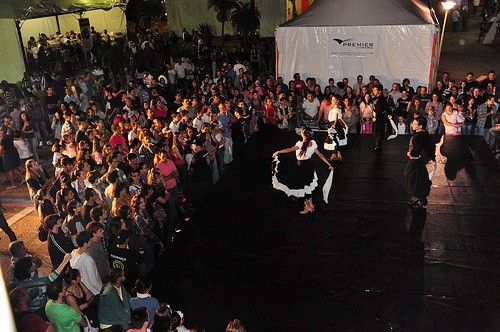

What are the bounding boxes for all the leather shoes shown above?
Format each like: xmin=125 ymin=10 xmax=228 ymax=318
xmin=409 ymin=196 xmax=427 ymax=209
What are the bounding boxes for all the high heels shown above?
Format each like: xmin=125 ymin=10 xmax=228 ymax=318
xmin=300 ymin=205 xmax=314 ymax=214
xmin=326 ymin=155 xmax=343 ymax=161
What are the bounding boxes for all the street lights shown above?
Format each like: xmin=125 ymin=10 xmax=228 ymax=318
xmin=440 ymin=0 xmax=460 ymax=59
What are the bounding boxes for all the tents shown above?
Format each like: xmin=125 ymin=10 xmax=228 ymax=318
xmin=0 ymin=0 xmax=128 ymax=83
xmin=272 ymin=0 xmax=441 ymax=94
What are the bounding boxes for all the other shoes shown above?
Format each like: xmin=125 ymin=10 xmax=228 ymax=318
xmin=371 ymin=145 xmax=382 ymax=151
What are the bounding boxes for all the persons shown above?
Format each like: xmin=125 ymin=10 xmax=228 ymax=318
xmin=271 ymin=127 xmax=335 ymax=215
xmin=0 ymin=0 xmax=500 ymax=332
